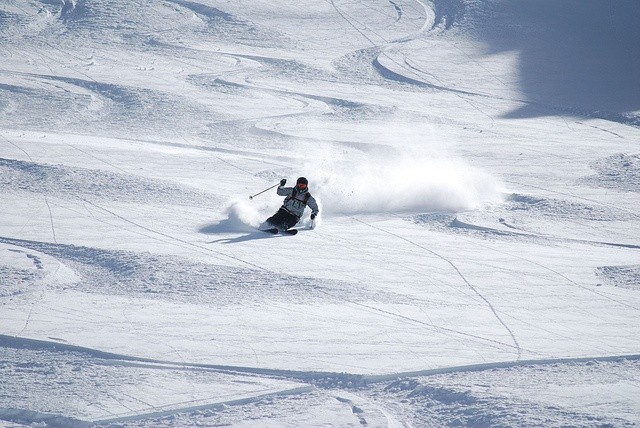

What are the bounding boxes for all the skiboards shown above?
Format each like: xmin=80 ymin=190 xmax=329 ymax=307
xmin=261 ymin=228 xmax=298 ymax=235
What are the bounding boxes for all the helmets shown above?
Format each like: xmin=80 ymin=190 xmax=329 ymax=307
xmin=297 ymin=177 xmax=308 ymax=190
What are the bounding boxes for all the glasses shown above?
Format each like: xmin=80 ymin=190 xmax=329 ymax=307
xmin=297 ymin=183 xmax=306 ymax=189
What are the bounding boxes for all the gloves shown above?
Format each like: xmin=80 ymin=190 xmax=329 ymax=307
xmin=280 ymin=179 xmax=286 ymax=186
xmin=311 ymin=214 xmax=315 ymax=219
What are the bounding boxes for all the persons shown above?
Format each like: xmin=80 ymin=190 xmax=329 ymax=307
xmin=264 ymin=177 xmax=320 ymax=231
